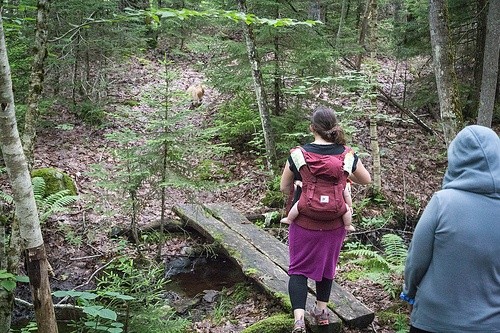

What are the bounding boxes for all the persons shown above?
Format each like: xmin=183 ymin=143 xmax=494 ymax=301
xmin=278 ymin=110 xmax=371 ymax=333
xmin=399 ymin=122 xmax=500 ymax=333
xmin=279 ymin=156 xmax=357 ymax=232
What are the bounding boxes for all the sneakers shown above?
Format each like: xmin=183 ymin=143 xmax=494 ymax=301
xmin=310 ymin=307 xmax=330 ymax=326
xmin=292 ymin=320 xmax=307 ymax=333
